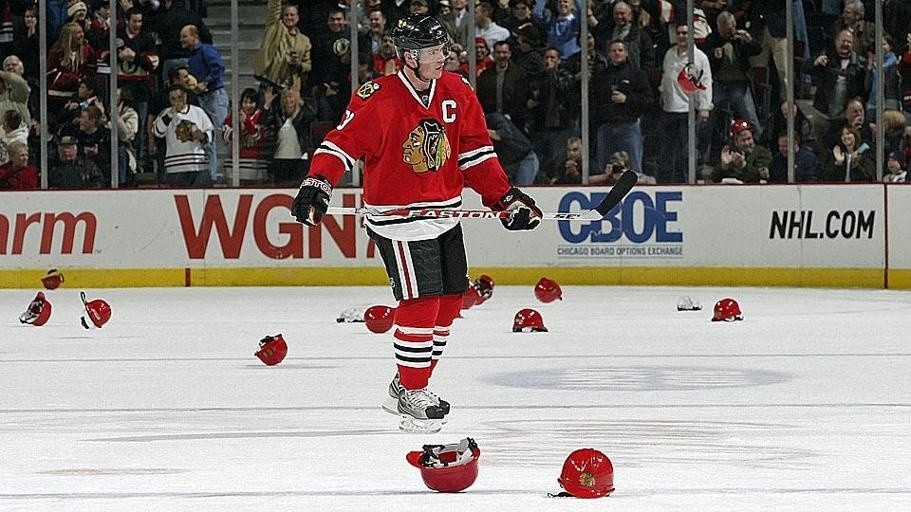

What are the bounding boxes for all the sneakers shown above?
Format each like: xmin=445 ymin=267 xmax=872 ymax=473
xmin=389 ymin=374 xmax=449 ymax=418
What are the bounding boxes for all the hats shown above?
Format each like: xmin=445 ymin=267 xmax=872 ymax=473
xmin=890 ymin=151 xmax=907 ymax=170
xmin=730 ymin=119 xmax=751 ymax=135
xmin=67 ymin=0 xmax=87 ymax=16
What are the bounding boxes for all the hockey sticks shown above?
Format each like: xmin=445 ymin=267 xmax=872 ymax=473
xmin=325 ymin=171 xmax=638 ymax=221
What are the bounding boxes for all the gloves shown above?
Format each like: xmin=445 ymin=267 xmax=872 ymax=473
xmin=290 ymin=176 xmax=332 ymax=226
xmin=494 ymin=188 xmax=542 ymax=230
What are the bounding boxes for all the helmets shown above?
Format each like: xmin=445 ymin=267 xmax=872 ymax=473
xmin=42 ymin=273 xmax=64 ymax=289
xmin=392 ymin=13 xmax=451 ymax=65
xmin=83 ymin=299 xmax=111 ymax=328
xmin=559 ymin=449 xmax=615 ymax=497
xmin=712 ymin=299 xmax=741 ymax=320
xmin=462 ymin=274 xmax=494 ymax=309
xmin=254 ymin=334 xmax=287 ymax=366
xmin=18 ymin=292 xmax=52 ymax=326
xmin=535 ymin=278 xmax=562 ymax=303
xmin=406 ymin=438 xmax=479 ymax=493
xmin=364 ymin=306 xmax=395 ymax=333
xmin=514 ymin=309 xmax=543 ymax=330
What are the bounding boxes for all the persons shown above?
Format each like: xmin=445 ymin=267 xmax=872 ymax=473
xmin=0 ymin=0 xmax=911 ymax=190
xmin=289 ymin=12 xmax=544 ymax=421
